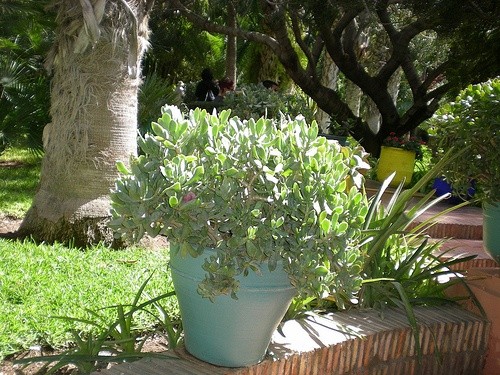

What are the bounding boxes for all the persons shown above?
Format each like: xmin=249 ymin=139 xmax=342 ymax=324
xmin=219 ymin=77 xmax=233 ymax=93
xmin=196 ymin=69 xmax=219 ymax=101
xmin=264 ymin=80 xmax=277 ymax=90
xmin=172 ymin=81 xmax=187 ymax=95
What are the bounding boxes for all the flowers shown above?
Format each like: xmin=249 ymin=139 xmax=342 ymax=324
xmin=387 ymin=132 xmax=426 ymax=163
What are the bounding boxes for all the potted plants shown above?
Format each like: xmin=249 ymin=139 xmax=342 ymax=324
xmin=417 ymin=75 xmax=500 ymax=261
xmin=107 ymin=103 xmax=372 ymax=369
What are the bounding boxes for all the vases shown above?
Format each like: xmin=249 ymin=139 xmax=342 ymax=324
xmin=377 ymin=146 xmax=416 ymax=188
